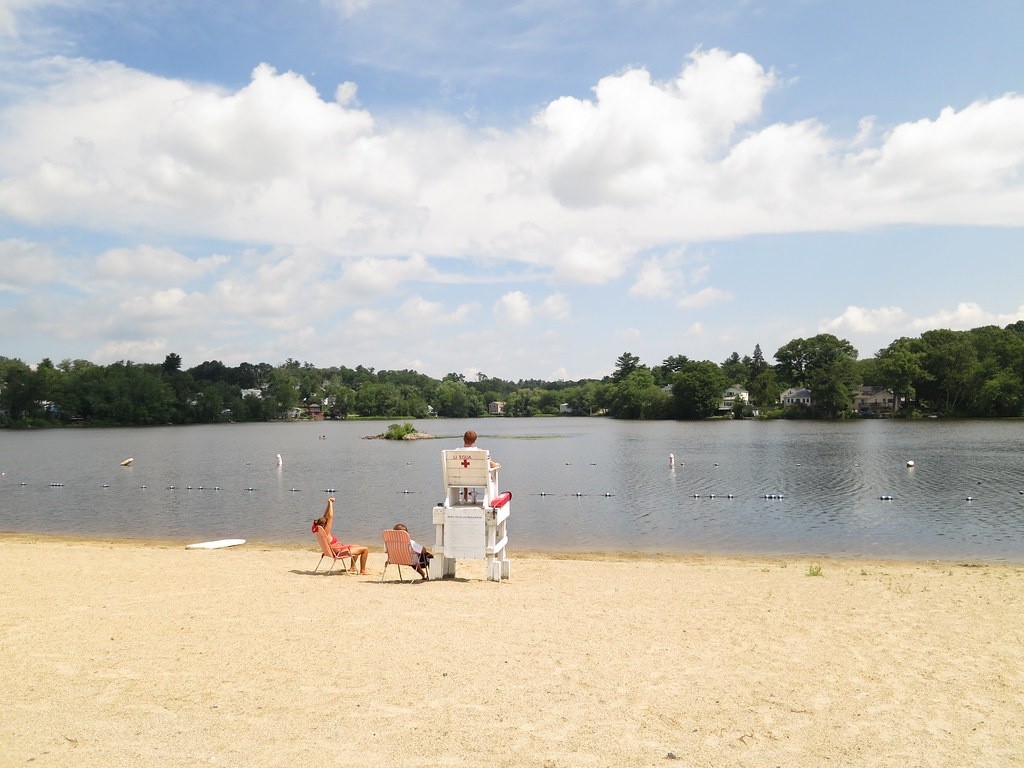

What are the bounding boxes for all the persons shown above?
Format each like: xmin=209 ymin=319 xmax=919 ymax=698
xmin=393 ymin=524 xmax=434 ymax=580
xmin=455 ymin=431 xmax=500 ymax=469
xmin=314 ymin=498 xmax=370 ymax=575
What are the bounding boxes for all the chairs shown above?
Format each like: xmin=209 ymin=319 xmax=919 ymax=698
xmin=313 ymin=525 xmax=358 ymax=576
xmin=441 ymin=449 xmax=501 ymax=508
xmin=379 ymin=529 xmax=430 ymax=585
